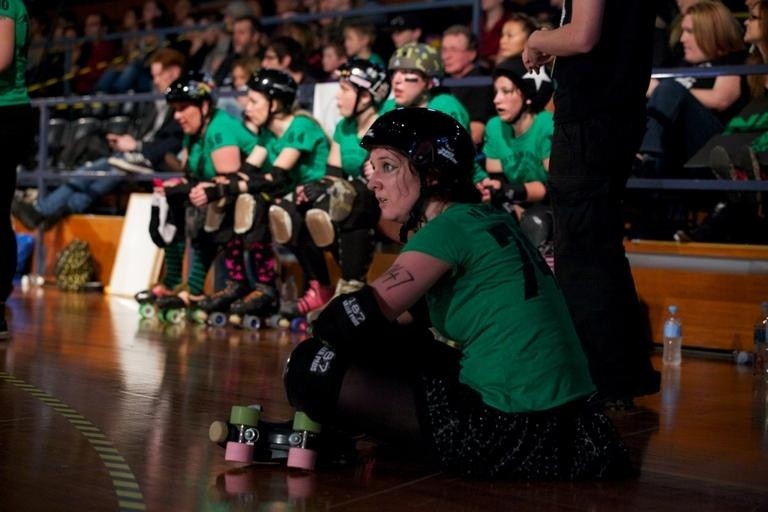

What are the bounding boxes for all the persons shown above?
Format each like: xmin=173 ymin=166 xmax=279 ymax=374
xmin=639 ymin=1 xmax=768 ymax=245
xmin=522 ymin=1 xmax=663 ymax=409
xmin=1 ymin=0 xmax=34 ymax=335
xmin=208 ymin=107 xmax=630 ymax=485
xmin=8 ymin=1 xmax=555 ymax=332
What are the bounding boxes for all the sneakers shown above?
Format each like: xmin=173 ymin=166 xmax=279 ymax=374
xmin=9 ymin=195 xmax=55 ymax=236
xmin=593 ymin=355 xmax=663 ymax=404
xmin=0 ymin=303 xmax=10 ymax=340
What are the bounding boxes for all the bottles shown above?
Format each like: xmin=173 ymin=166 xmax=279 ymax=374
xmin=662 ymin=304 xmax=683 ymax=366
xmin=749 ymin=302 xmax=768 ymax=451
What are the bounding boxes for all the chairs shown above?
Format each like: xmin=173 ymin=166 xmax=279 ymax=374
xmin=1 ymin=0 xmax=768 ymax=292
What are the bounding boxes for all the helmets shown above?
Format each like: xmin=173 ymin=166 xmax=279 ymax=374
xmin=331 ymin=56 xmax=392 ymax=105
xmin=385 ymin=42 xmax=447 ymax=84
xmin=245 ymin=68 xmax=302 ymax=108
xmin=163 ymin=72 xmax=222 ymax=106
xmin=358 ymin=105 xmax=481 ymax=186
xmin=489 ymin=50 xmax=556 ymax=114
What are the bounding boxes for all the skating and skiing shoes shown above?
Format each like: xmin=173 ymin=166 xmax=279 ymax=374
xmin=204 ymin=403 xmax=333 ymax=476
xmin=131 ymin=282 xmax=339 ymax=338
